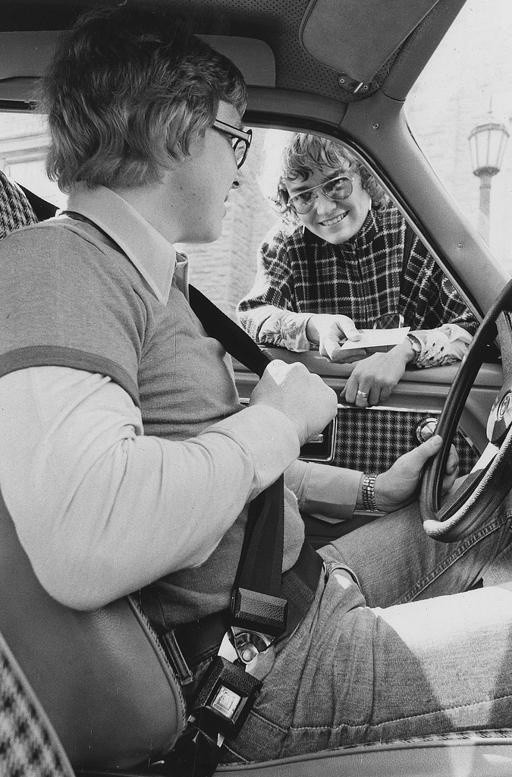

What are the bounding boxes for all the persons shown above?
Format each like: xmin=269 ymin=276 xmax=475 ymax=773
xmin=234 ymin=129 xmax=500 ymax=407
xmin=0 ymin=12 xmax=512 ymax=770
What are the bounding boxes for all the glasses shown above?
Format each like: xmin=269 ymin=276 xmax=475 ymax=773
xmin=283 ymin=175 xmax=354 ymax=215
xmin=202 ymin=114 xmax=256 ymax=171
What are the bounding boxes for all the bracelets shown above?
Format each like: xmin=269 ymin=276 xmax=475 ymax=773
xmin=362 ymin=472 xmax=378 ymax=515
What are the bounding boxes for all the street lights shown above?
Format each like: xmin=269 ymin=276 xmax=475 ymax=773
xmin=467 ymin=95 xmax=510 ymax=246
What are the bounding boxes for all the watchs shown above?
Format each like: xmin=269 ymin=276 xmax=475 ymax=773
xmin=406 ymin=335 xmax=422 ymax=369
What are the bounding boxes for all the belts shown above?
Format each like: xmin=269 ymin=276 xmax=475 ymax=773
xmin=144 ymin=608 xmax=234 ymax=674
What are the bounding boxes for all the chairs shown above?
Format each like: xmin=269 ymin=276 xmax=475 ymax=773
xmin=0 ymin=175 xmax=510 ymax=772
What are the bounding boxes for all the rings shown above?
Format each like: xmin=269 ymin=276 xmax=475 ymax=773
xmin=355 ymin=389 xmax=370 ymax=400
